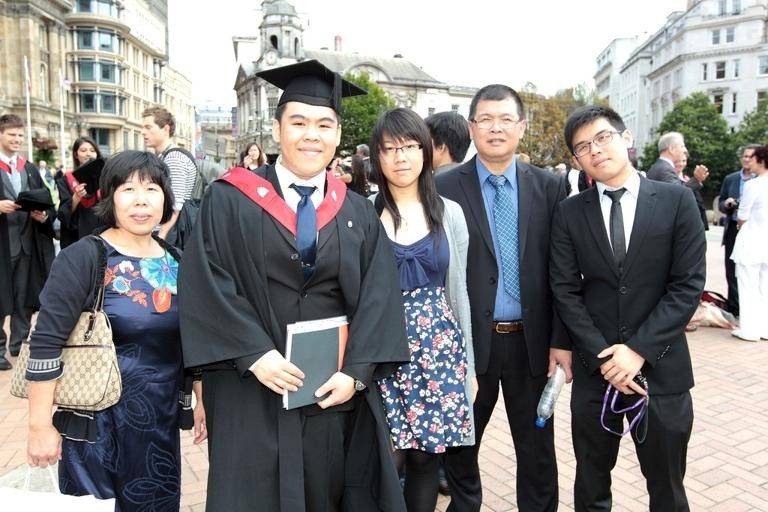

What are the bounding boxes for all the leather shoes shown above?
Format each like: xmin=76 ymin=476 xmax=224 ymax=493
xmin=0 ymin=355 xmax=11 ymax=372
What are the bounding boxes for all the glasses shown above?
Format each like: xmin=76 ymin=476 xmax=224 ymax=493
xmin=382 ymin=143 xmax=424 ymax=155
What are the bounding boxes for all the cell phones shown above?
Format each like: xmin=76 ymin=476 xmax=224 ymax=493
xmin=635 ymin=376 xmax=647 ymax=389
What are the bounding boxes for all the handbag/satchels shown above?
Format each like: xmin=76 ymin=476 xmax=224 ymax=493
xmin=8 ymin=309 xmax=123 ymax=414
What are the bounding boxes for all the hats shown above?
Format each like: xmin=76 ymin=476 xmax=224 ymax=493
xmin=14 ymin=188 xmax=56 ymax=213
xmin=70 ymin=157 xmax=105 ymax=198
xmin=253 ymin=57 xmax=368 ymax=109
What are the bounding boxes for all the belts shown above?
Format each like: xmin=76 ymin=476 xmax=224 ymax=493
xmin=492 ymin=320 xmax=524 ymax=334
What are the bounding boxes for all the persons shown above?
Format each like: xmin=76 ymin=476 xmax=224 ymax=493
xmin=0 ymin=59 xmax=768 ymax=511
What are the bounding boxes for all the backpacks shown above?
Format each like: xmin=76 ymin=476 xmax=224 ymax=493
xmin=177 ymin=169 xmax=209 ymax=235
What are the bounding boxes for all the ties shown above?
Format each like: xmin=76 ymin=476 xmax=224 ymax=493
xmin=288 ymin=183 xmax=317 ymax=277
xmin=603 ymin=188 xmax=630 ymax=264
xmin=8 ymin=160 xmax=22 ymax=197
xmin=485 ymin=176 xmax=524 ymax=302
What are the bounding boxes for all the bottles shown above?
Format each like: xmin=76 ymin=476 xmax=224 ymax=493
xmin=536 ymin=364 xmax=566 ymax=428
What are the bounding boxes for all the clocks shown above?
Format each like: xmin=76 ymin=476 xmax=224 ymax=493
xmin=265 ymin=48 xmax=278 ymax=65
